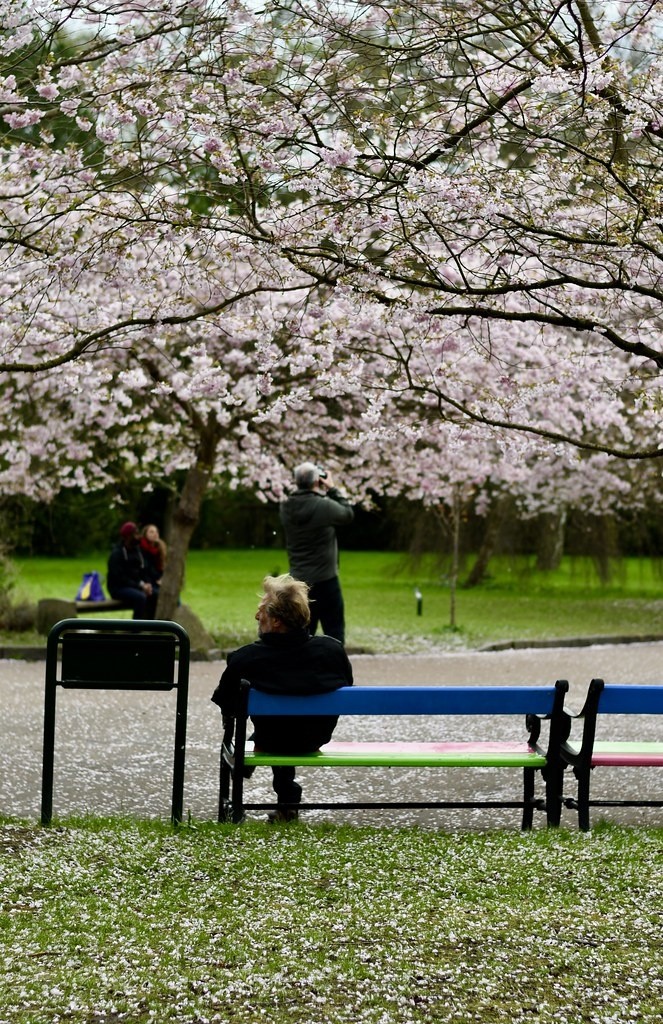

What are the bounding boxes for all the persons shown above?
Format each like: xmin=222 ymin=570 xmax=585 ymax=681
xmin=211 ymin=572 xmax=355 ymax=824
xmin=279 ymin=461 xmax=356 ymax=648
xmin=106 ymin=522 xmax=180 ymax=620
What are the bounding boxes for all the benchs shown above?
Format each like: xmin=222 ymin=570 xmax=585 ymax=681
xmin=37 ymin=596 xmax=131 ymax=635
xmin=564 ymin=678 xmax=663 ymax=832
xmin=219 ymin=680 xmax=569 ymax=831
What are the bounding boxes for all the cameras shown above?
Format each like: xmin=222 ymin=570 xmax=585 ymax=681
xmin=316 ymin=465 xmax=327 ymax=486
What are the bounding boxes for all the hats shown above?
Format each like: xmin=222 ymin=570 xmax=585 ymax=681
xmin=120 ymin=521 xmax=137 ymax=537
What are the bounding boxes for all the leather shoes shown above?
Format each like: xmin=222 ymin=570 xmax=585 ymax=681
xmin=242 ymin=765 xmax=255 ymax=778
xmin=273 ymin=782 xmax=303 ymax=815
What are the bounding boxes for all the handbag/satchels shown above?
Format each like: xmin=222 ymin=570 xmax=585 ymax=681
xmin=75 ymin=573 xmax=106 ymax=601
